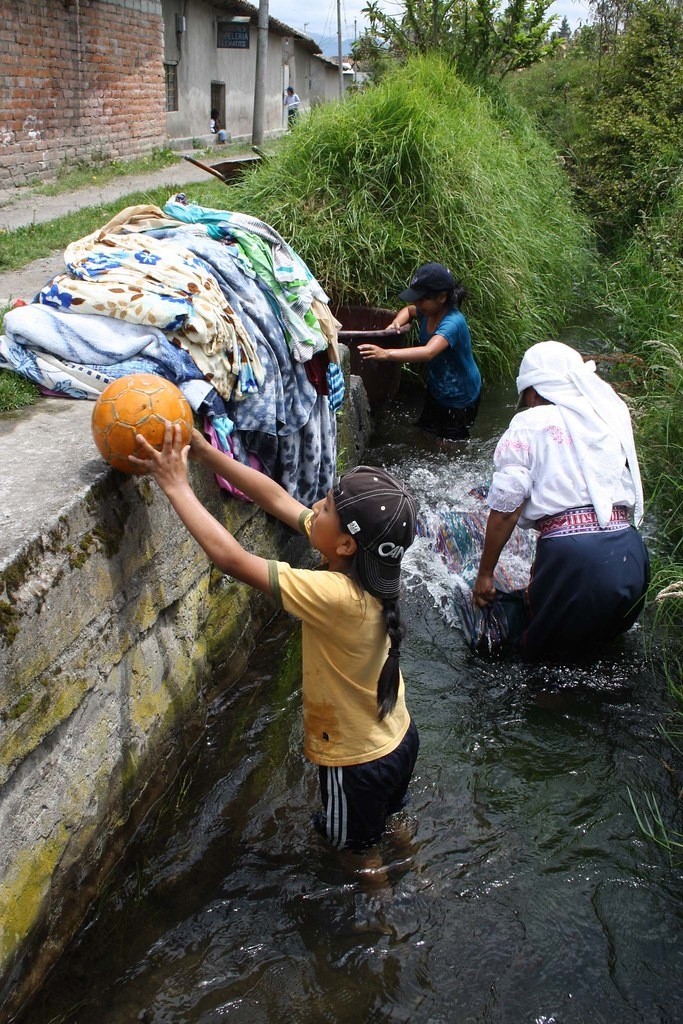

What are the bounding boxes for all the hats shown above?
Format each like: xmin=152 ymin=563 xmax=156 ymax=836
xmin=397 ymin=263 xmax=454 ymax=302
xmin=332 ymin=465 xmax=417 ymax=598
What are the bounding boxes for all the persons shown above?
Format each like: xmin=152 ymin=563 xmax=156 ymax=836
xmin=284 ymin=86 xmax=300 ymax=131
xmin=358 ymin=262 xmax=483 ymax=423
xmin=211 ymin=108 xmax=228 ymax=144
xmin=472 ymin=340 xmax=649 ymax=645
xmin=127 ymin=420 xmax=418 ymax=849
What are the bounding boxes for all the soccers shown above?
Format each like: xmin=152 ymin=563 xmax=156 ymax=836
xmin=90 ymin=372 xmax=195 ymax=477
